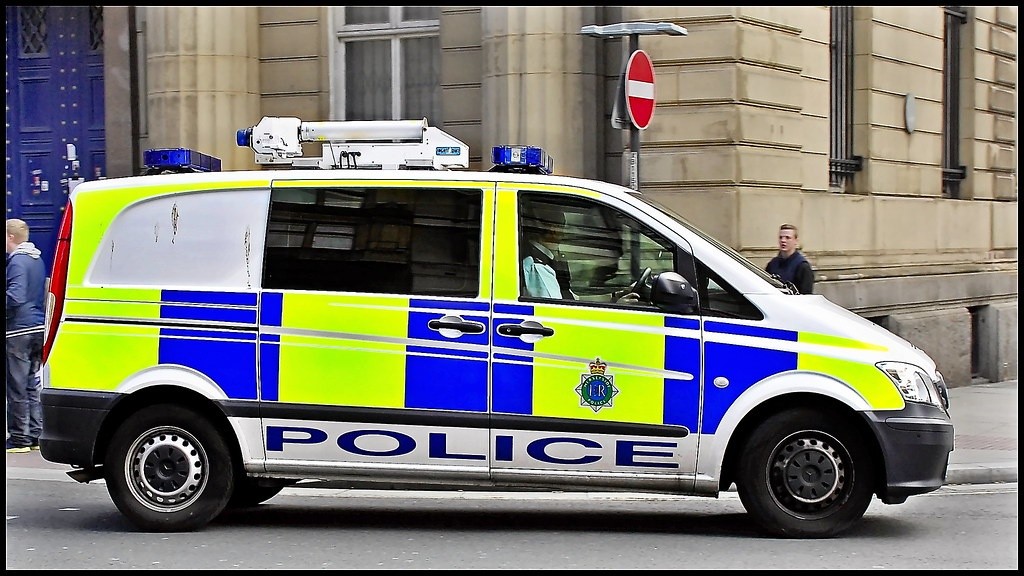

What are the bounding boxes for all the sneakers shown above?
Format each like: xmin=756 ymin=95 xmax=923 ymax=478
xmin=6 ymin=440 xmax=30 ymax=453
xmin=31 ymin=442 xmax=39 ymax=450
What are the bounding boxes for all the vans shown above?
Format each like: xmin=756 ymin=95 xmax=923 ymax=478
xmin=35 ymin=119 xmax=954 ymax=537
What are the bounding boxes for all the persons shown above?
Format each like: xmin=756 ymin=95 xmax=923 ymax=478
xmin=767 ymin=224 xmax=813 ymax=294
xmin=6 ymin=219 xmax=46 ymax=451
xmin=522 ymin=209 xmax=652 ymax=304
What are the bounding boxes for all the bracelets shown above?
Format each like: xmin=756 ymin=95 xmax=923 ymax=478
xmin=612 ymin=292 xmax=615 ymax=298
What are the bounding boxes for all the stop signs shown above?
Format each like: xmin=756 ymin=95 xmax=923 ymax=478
xmin=625 ymin=49 xmax=657 ymax=131
